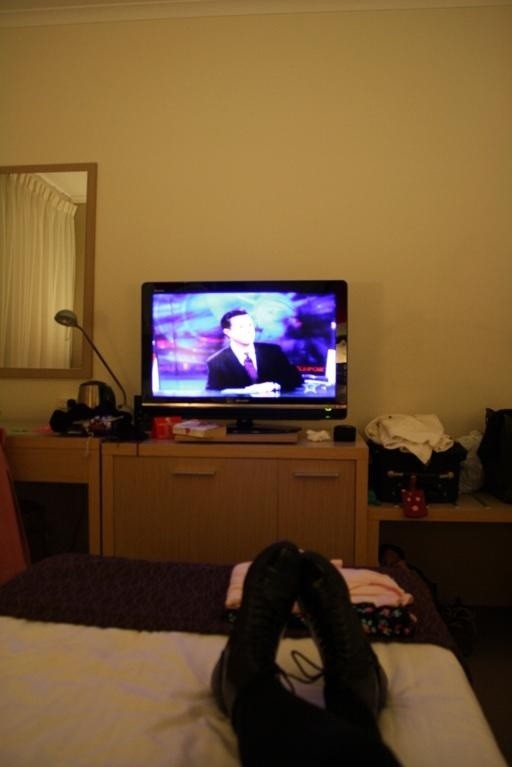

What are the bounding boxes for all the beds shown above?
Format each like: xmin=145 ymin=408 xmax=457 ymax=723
xmin=0 ymin=550 xmax=510 ymax=767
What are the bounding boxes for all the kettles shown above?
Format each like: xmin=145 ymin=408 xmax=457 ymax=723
xmin=76 ymin=380 xmax=116 ymax=411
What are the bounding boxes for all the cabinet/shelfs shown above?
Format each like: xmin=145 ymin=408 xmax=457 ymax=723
xmin=100 ymin=428 xmax=369 ymax=570
xmin=369 ymin=491 xmax=507 ymax=611
xmin=2 ymin=424 xmax=100 ymax=560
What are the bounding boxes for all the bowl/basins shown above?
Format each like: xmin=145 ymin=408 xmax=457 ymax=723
xmin=333 ymin=424 xmax=356 ymax=443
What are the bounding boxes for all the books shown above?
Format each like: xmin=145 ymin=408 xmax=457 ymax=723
xmin=173 ymin=419 xmax=227 ymax=438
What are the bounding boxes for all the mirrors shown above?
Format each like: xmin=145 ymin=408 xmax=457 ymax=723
xmin=0 ymin=161 xmax=97 ymax=379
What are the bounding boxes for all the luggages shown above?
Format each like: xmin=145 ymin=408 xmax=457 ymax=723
xmin=367 ymin=441 xmax=468 ymax=504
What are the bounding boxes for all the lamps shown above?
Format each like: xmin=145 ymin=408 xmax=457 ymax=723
xmin=54 ymin=310 xmax=134 ymax=416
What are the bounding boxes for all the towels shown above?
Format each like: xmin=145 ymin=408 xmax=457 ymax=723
xmin=364 ymin=413 xmax=455 ymax=465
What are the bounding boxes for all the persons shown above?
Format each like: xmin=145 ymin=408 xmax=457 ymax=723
xmin=205 ymin=309 xmax=305 ymax=394
xmin=211 ymin=540 xmax=405 ymax=767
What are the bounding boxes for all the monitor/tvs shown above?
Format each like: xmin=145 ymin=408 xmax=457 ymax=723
xmin=141 ymin=279 xmax=348 ymax=435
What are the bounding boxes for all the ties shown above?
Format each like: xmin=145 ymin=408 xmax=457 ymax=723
xmin=244 ymin=353 xmax=257 ymax=380
